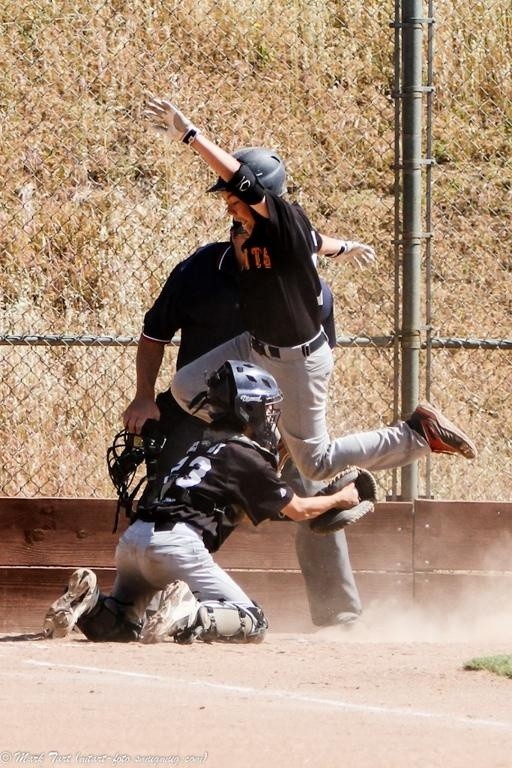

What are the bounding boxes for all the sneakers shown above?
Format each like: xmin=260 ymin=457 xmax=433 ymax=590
xmin=141 ymin=581 xmax=199 ymax=642
xmin=407 ymin=405 xmax=476 ymax=459
xmin=43 ymin=568 xmax=99 ymax=639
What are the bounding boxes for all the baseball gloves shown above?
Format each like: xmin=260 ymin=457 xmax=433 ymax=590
xmin=309 ymin=465 xmax=376 ymax=533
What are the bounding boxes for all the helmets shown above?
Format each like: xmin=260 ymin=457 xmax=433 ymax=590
xmin=107 ymin=421 xmax=167 ymax=506
xmin=206 ymin=149 xmax=288 ymax=198
xmin=207 ymin=360 xmax=284 ymax=450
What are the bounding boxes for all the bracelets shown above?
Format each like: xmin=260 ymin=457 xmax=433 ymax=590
xmin=179 ymin=126 xmax=201 ymax=143
xmin=326 ymin=236 xmax=348 ymax=259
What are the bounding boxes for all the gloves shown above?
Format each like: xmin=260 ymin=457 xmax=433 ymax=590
xmin=328 ymin=239 xmax=376 ymax=271
xmin=146 ymin=98 xmax=195 ymax=145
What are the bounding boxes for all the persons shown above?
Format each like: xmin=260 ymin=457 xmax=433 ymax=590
xmin=119 ymin=216 xmax=369 ymax=629
xmin=143 ymin=95 xmax=477 ymax=478
xmin=46 ymin=360 xmax=358 ymax=642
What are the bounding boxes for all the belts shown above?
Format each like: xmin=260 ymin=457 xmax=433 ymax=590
xmin=252 ymin=335 xmax=326 ymax=358
xmin=154 ymin=523 xmax=177 ymax=531
